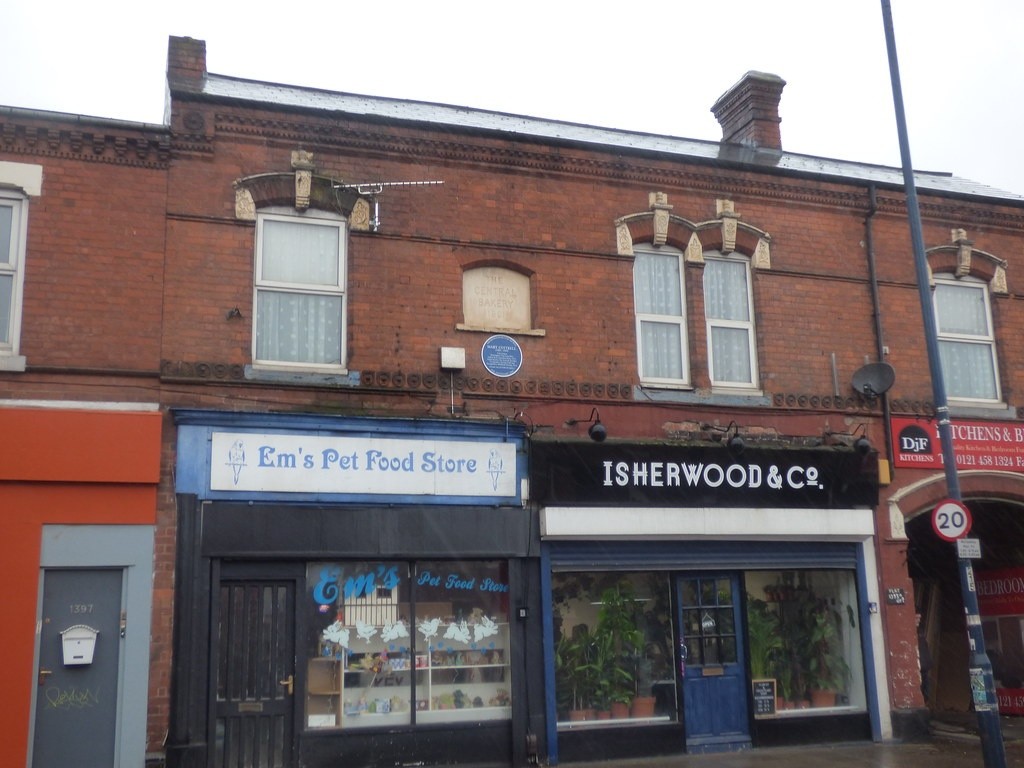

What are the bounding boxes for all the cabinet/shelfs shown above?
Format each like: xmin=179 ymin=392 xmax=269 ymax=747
xmin=306 ymin=623 xmax=509 ymax=729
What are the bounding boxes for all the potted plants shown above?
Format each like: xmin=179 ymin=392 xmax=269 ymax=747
xmin=709 ymin=569 xmax=853 ymax=715
xmin=551 ymin=571 xmax=673 ymax=726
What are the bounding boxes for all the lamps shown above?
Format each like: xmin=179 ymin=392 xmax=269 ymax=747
xmin=570 ymin=407 xmax=607 ymax=442
xmin=702 ymin=420 xmax=744 ymax=452
xmin=825 ymin=422 xmax=871 ymax=458
